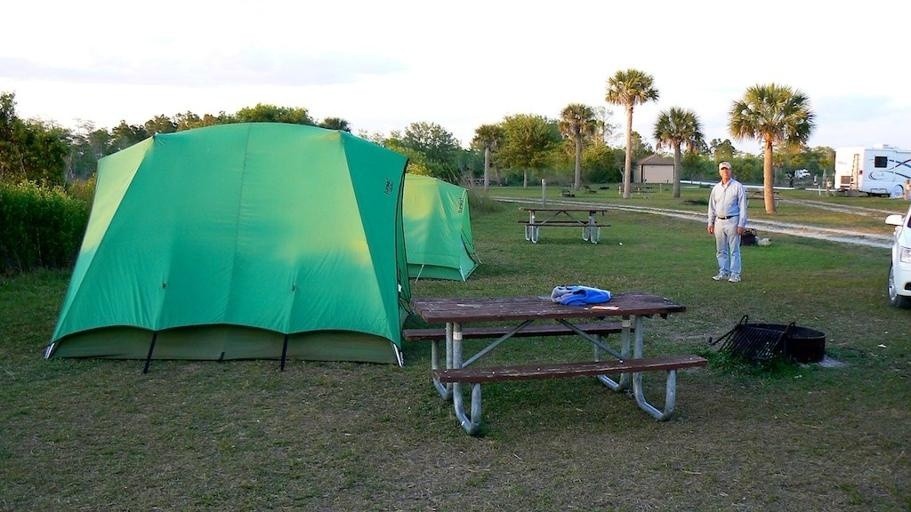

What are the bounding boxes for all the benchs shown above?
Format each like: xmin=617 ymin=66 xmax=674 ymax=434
xmin=517 ymin=220 xmax=598 ymax=241
xmin=432 ymin=354 xmax=708 ymax=435
xmin=402 ymin=321 xmax=637 ymax=401
xmin=746 ymin=197 xmax=782 ymax=208
xmin=618 ymin=183 xmax=663 ymax=197
xmin=525 ymin=223 xmax=611 ymax=245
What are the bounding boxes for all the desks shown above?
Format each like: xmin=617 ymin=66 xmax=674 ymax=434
xmin=411 ymin=294 xmax=686 ymax=416
xmin=519 ymin=207 xmax=608 ymax=241
xmin=745 ymin=190 xmax=780 ymax=205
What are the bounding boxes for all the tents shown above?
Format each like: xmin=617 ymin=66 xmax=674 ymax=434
xmin=40 ymin=121 xmax=412 ymax=376
xmin=400 ymin=169 xmax=484 ymax=282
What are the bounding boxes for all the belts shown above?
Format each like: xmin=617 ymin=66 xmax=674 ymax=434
xmin=717 ymin=216 xmax=733 ymax=220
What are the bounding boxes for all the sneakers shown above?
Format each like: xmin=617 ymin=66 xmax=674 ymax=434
xmin=712 ymin=274 xmax=728 ymax=280
xmin=728 ymin=276 xmax=742 ymax=282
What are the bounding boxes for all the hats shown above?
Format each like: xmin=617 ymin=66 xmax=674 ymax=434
xmin=719 ymin=162 xmax=732 ymax=170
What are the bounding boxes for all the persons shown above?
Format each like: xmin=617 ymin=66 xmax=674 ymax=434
xmin=706 ymin=163 xmax=749 ymax=284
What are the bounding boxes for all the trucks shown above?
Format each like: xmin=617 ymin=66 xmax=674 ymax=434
xmin=833 ymin=148 xmax=911 ymax=201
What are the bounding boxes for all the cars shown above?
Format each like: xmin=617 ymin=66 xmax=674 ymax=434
xmin=882 ymin=204 xmax=911 ymax=309
